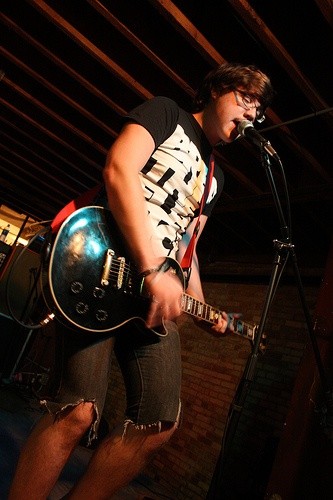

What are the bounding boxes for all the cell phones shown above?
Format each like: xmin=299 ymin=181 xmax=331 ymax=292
xmin=237 ymin=120 xmax=280 ymax=161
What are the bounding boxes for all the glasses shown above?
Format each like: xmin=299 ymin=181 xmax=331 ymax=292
xmin=233 ymin=87 xmax=266 ymax=124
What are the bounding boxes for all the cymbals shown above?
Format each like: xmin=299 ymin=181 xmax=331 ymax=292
xmin=24 ymin=220 xmax=52 ymax=227
xmin=20 ymin=225 xmax=45 ymax=240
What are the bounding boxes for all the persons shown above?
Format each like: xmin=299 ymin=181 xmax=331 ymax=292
xmin=9 ymin=66 xmax=271 ymax=500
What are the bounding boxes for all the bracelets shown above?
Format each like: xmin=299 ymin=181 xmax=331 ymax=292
xmin=138 ymin=259 xmax=171 ymax=278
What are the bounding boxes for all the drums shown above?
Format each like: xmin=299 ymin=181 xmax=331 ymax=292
xmin=6 ymin=226 xmax=56 ymax=329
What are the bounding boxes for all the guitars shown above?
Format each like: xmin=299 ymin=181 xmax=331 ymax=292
xmin=40 ymin=205 xmax=268 ymax=351
